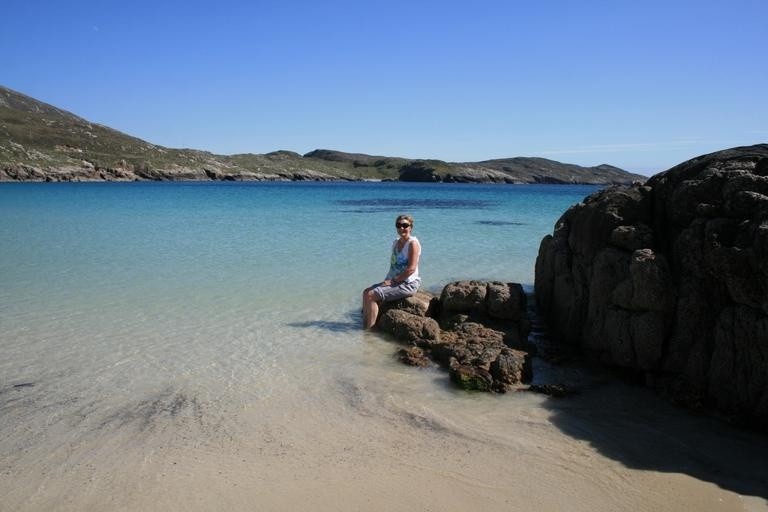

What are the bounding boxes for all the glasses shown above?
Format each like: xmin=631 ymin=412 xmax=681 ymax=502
xmin=396 ymin=223 xmax=411 ymax=228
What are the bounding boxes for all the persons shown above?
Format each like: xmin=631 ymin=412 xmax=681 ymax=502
xmin=363 ymin=215 xmax=421 ymax=329
